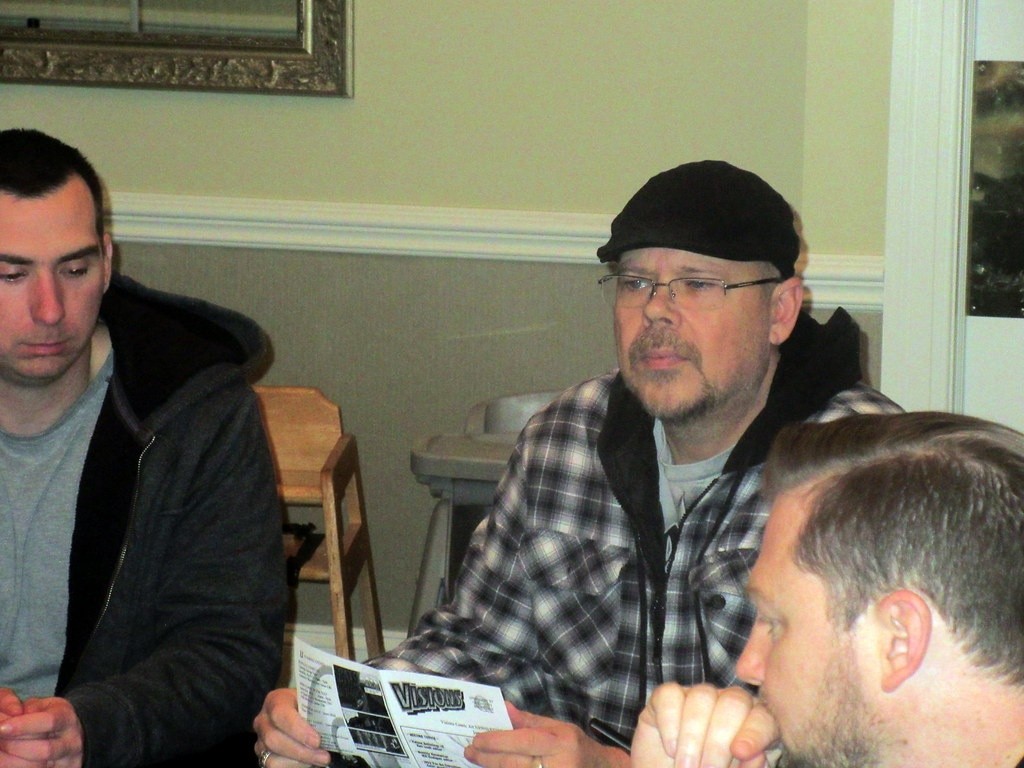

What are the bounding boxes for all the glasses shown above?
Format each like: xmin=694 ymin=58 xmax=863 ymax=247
xmin=598 ymin=273 xmax=782 ymax=308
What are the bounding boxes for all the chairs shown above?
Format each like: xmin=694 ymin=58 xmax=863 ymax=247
xmin=407 ymin=389 xmax=566 ymax=637
xmin=252 ymin=385 xmax=388 ymax=682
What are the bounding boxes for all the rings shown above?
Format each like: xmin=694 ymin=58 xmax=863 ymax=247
xmin=534 ymin=757 xmax=543 ymax=768
xmin=259 ymin=750 xmax=272 ymax=768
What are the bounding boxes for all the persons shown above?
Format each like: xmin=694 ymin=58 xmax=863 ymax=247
xmin=631 ymin=411 xmax=1024 ymax=768
xmin=253 ymin=160 xmax=906 ymax=768
xmin=0 ymin=129 xmax=287 ymax=768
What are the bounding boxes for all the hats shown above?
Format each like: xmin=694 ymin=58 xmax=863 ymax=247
xmin=597 ymin=159 xmax=800 ymax=279
